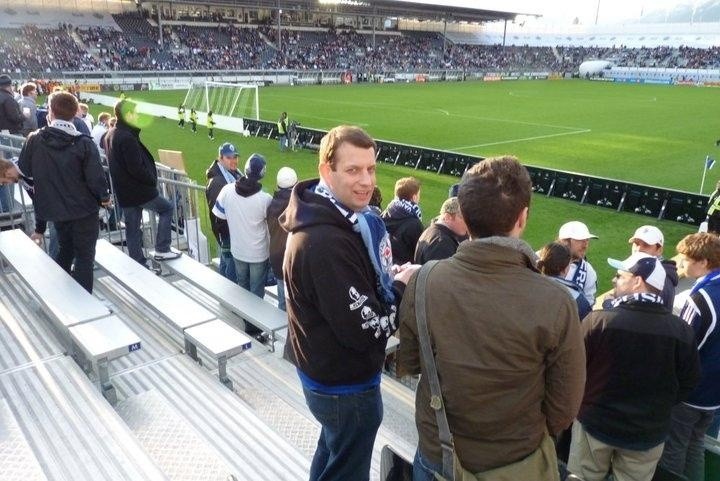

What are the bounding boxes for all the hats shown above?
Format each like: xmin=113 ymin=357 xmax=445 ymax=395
xmin=559 ymin=220 xmax=598 ymax=243
xmin=606 ymin=250 xmax=667 ymax=291
xmin=276 ymin=166 xmax=298 ymax=192
xmin=218 ymin=142 xmax=240 ymax=160
xmin=243 ymin=154 xmax=266 ymax=178
xmin=629 ymin=223 xmax=664 ymax=251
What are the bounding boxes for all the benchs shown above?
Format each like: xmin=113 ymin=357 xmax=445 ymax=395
xmin=0 ymin=228 xmax=142 ymax=402
xmin=12 ymin=175 xmax=163 ymax=237
xmin=93 ymin=238 xmax=253 ymax=394
xmin=148 ymin=245 xmax=289 ymax=354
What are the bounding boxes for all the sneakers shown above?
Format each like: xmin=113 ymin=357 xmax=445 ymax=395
xmin=151 ymin=267 xmax=161 ymax=275
xmin=153 ymin=250 xmax=183 ymax=261
